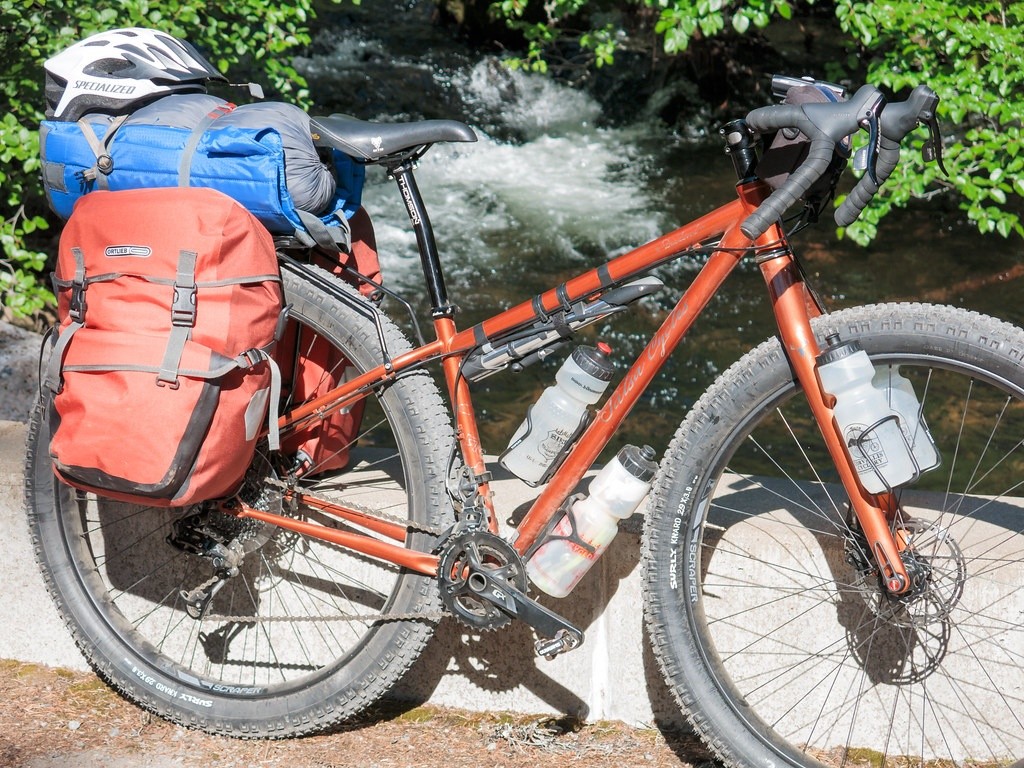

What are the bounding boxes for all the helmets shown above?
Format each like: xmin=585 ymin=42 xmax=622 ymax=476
xmin=44 ymin=26 xmax=228 ymax=124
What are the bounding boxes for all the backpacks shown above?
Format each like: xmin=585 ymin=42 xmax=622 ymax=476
xmin=43 ymin=186 xmax=294 ymax=509
xmin=270 ymin=203 xmax=382 ymax=476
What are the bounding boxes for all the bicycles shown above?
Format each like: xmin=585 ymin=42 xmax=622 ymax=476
xmin=24 ymin=73 xmax=1024 ymax=768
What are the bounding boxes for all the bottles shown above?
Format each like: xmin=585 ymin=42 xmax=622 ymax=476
xmin=870 ymin=365 xmax=940 ymax=474
xmin=812 ymin=331 xmax=915 ymax=494
xmin=501 ymin=340 xmax=617 ymax=484
xmin=523 ymin=444 xmax=659 ymax=600
